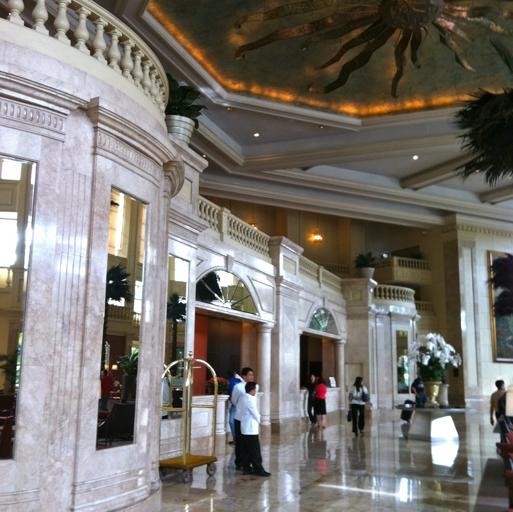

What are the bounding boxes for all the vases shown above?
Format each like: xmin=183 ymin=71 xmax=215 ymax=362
xmin=423 ymin=381 xmax=442 ymax=408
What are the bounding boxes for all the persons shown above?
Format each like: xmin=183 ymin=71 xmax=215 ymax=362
xmin=311 ymin=378 xmax=327 ymax=429
xmin=400 ymin=378 xmax=428 ymax=423
xmin=238 ymin=380 xmax=271 ymax=477
xmin=489 ymin=378 xmax=507 ymax=427
xmin=226 ymin=370 xmax=246 ymax=470
xmin=230 ymin=368 xmax=257 ymax=475
xmin=303 ymin=374 xmax=318 ymax=428
xmin=349 ymin=375 xmax=368 ymax=436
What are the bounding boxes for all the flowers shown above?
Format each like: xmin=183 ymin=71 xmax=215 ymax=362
xmin=397 ymin=332 xmax=462 ymax=382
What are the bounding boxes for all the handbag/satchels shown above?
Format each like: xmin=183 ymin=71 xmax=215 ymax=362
xmin=347 ymin=410 xmax=352 ymax=422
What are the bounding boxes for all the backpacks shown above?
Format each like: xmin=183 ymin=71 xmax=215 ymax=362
xmin=361 ymin=385 xmax=371 ymax=402
